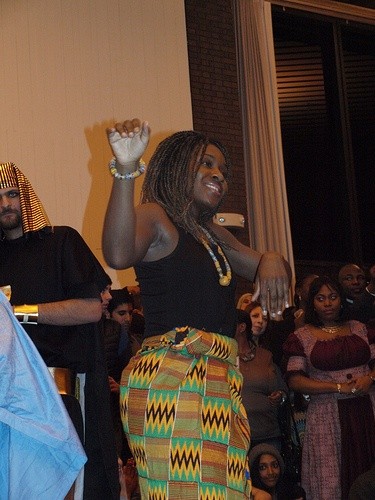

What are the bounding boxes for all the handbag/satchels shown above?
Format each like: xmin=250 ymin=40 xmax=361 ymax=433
xmin=276 ymin=436 xmax=303 ymax=487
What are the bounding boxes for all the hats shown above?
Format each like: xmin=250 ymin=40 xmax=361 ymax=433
xmin=249 ymin=444 xmax=284 ymax=475
